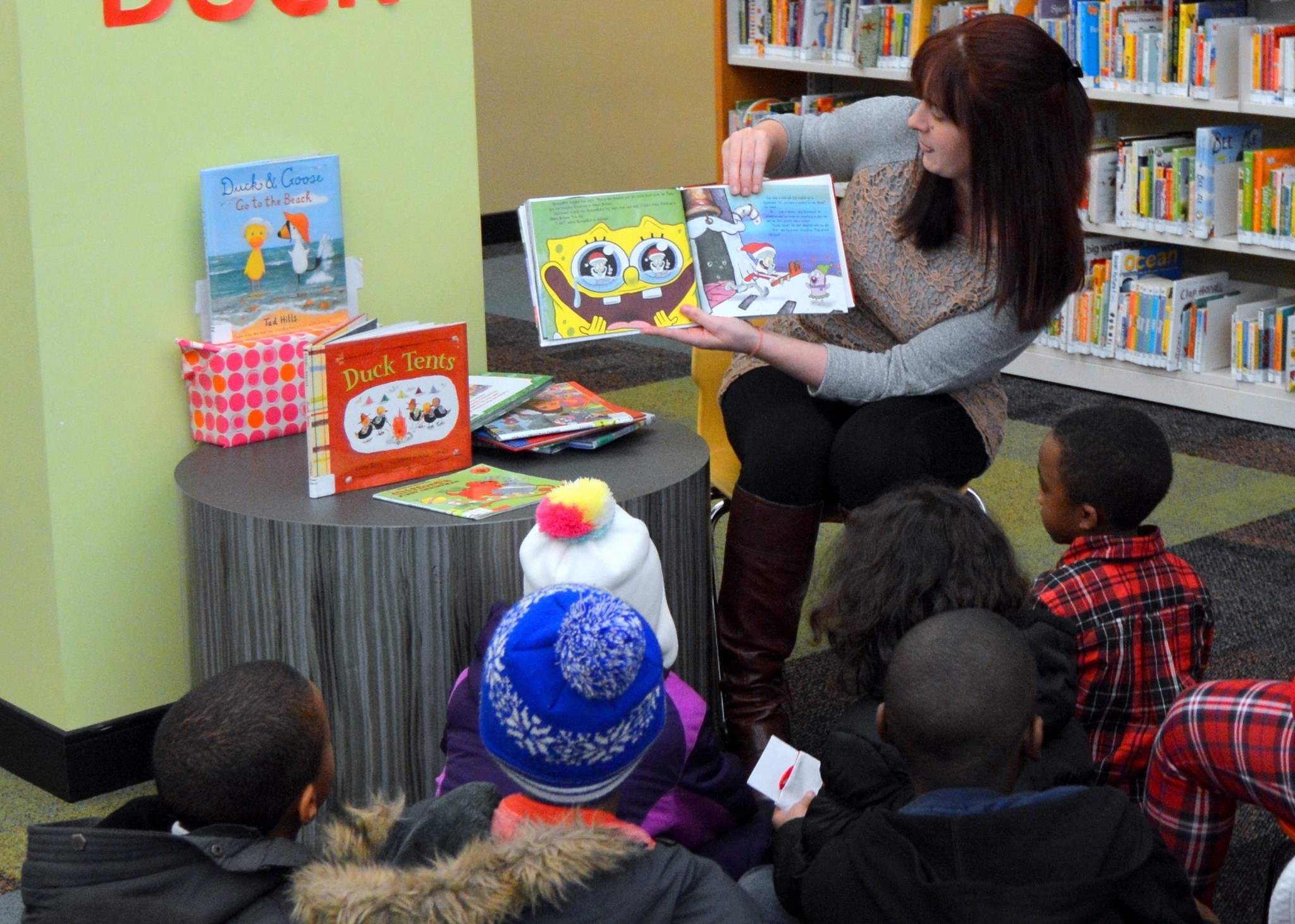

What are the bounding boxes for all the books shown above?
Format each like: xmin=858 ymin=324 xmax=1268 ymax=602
xmin=303 ymin=310 xmax=473 ymax=499
xmin=371 ymin=460 xmax=570 ymax=522
xmin=516 ymin=172 xmax=858 ymax=348
xmin=724 ymin=1 xmax=1294 ymax=392
xmin=198 ymin=153 xmax=351 ymax=342
xmin=467 ymin=369 xmax=656 ymax=455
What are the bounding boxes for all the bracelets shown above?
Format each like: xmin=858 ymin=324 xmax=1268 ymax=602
xmin=747 ymin=325 xmax=763 ymax=361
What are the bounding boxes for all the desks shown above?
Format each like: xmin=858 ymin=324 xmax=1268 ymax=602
xmin=173 ymin=412 xmax=716 ymax=827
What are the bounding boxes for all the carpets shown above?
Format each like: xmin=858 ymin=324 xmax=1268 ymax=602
xmin=796 ymin=499 xmax=1294 ymax=924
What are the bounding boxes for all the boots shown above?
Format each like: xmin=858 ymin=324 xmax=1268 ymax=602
xmin=716 ymin=481 xmax=820 ymax=805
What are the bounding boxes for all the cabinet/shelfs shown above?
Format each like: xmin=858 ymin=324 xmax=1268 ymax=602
xmin=708 ymin=0 xmax=1291 ymax=430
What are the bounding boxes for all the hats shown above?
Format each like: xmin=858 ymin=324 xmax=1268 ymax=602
xmin=477 ymin=584 xmax=669 ymax=805
xmin=519 ymin=477 xmax=680 ymax=670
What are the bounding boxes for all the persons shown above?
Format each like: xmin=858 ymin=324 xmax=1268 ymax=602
xmin=763 ymin=482 xmax=1095 ymax=921
xmin=436 ymin=478 xmax=776 ymax=878
xmin=285 ymin=583 xmax=766 ymax=923
xmin=1148 ymin=677 xmax=1295 ymax=924
xmin=20 ymin=655 xmax=337 ymax=924
xmin=634 ymin=10 xmax=1093 ymax=771
xmin=804 ymin=606 xmax=1205 ymax=924
xmin=1024 ymin=404 xmax=1213 ymax=808
